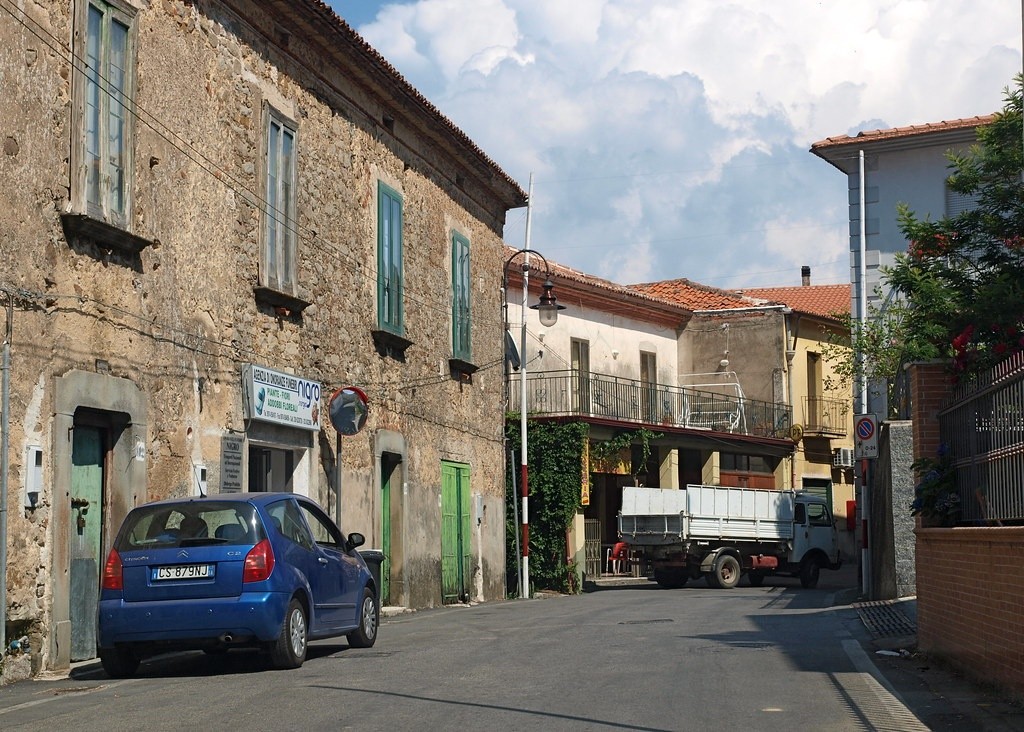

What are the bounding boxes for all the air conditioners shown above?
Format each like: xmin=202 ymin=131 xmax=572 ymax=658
xmin=834 ymin=447 xmax=855 ymax=466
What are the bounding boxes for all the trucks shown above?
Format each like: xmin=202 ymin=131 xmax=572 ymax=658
xmin=616 ymin=483 xmax=842 ymax=590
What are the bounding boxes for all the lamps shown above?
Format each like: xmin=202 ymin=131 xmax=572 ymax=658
xmin=504 ymin=250 xmax=566 ymax=326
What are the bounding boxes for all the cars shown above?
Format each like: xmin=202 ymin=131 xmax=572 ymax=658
xmin=93 ymin=493 xmax=381 ymax=670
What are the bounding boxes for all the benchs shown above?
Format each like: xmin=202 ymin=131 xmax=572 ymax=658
xmin=681 ymin=402 xmax=740 ymax=433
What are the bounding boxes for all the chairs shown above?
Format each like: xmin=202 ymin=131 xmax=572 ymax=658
xmin=607 ymin=541 xmax=629 ymax=576
